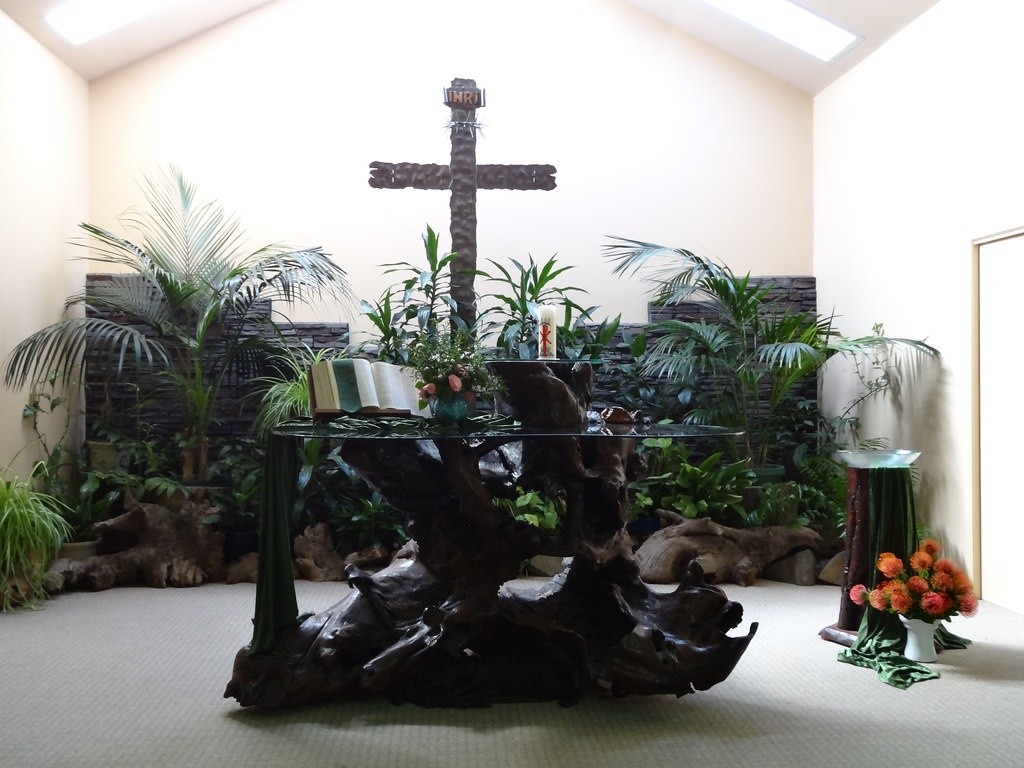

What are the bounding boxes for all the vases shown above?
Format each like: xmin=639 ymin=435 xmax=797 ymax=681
xmin=433 ymin=381 xmax=469 ymax=421
xmin=898 ymin=614 xmax=941 ymax=663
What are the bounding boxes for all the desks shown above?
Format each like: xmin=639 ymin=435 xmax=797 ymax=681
xmin=224 ymin=357 xmax=760 ymax=709
xmin=818 ymin=448 xmax=921 ymax=647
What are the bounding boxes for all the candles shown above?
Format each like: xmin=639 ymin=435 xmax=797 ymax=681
xmin=538 ymin=305 xmax=556 ymax=359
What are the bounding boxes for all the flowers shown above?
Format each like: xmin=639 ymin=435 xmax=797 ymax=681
xmin=850 ymin=540 xmax=978 ymax=623
xmin=409 ymin=317 xmax=512 ymax=411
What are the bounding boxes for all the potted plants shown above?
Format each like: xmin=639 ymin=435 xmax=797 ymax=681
xmin=0 ymin=158 xmax=942 ymax=614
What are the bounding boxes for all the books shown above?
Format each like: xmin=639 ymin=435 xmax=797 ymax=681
xmin=311 ymin=359 xmax=433 ymax=419
